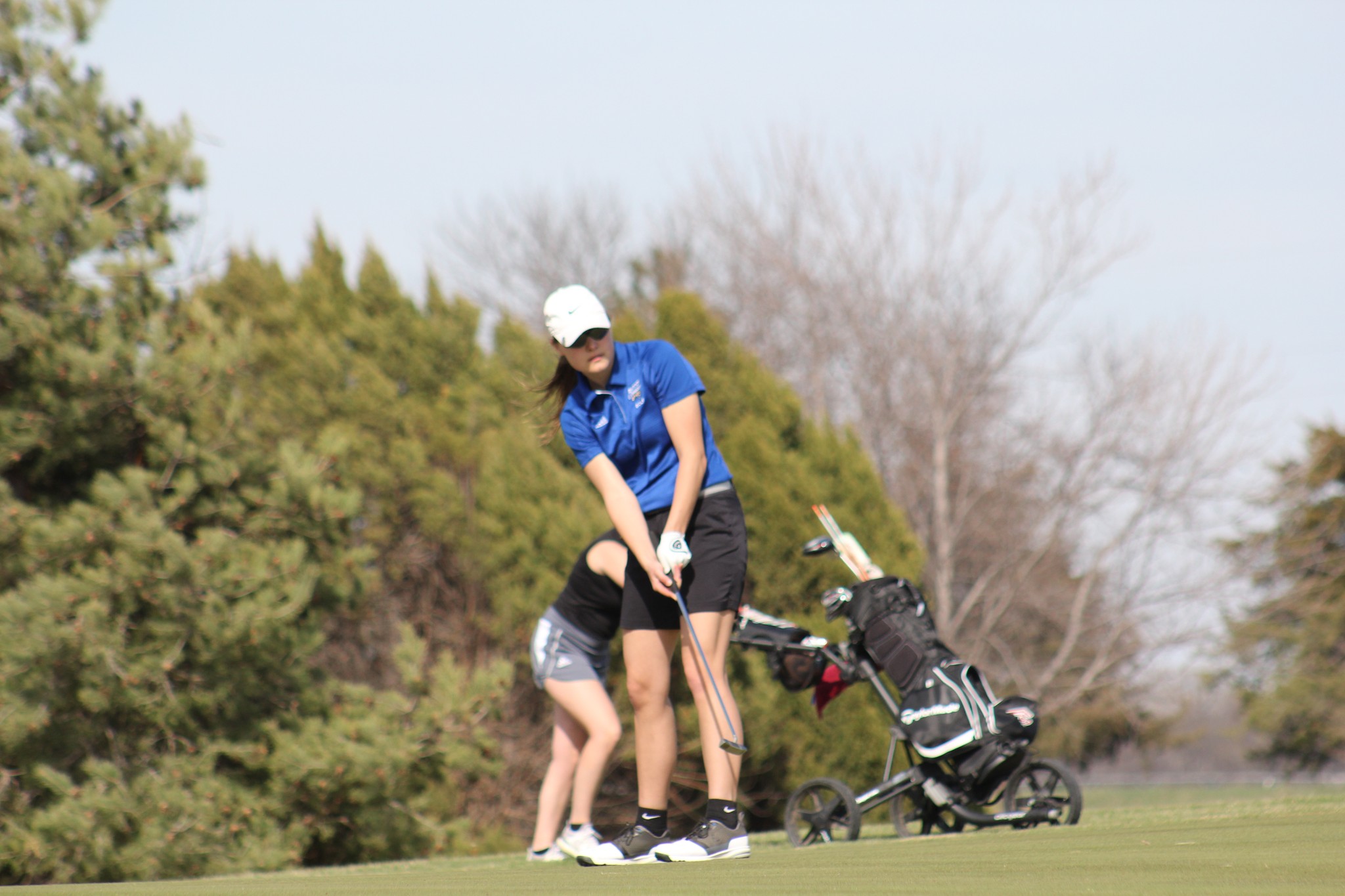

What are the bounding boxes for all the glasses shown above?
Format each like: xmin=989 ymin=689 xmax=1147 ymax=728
xmin=572 ymin=328 xmax=609 ymax=347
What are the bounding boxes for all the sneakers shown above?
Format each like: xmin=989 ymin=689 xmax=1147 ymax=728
xmin=576 ymin=825 xmax=669 ymax=866
xmin=655 ymin=812 xmax=750 ymax=862
xmin=526 ymin=843 xmax=569 ymax=861
xmin=556 ymin=823 xmax=599 ymax=858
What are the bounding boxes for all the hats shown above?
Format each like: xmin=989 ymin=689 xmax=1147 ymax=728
xmin=544 ymin=285 xmax=611 ymax=347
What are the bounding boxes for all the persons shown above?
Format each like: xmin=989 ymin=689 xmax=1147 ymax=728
xmin=528 ymin=526 xmax=628 ymax=860
xmin=522 ymin=286 xmax=749 ymax=866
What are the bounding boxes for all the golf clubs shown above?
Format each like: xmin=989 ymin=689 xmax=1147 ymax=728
xmin=663 ymin=565 xmax=752 ymax=758
xmin=806 ymin=537 xmax=856 ymax=613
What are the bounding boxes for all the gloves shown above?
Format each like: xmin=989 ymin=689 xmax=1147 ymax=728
xmin=656 ymin=532 xmax=693 ymax=576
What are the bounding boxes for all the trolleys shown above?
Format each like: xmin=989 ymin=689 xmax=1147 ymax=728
xmin=733 ymin=619 xmax=1086 ymax=847
